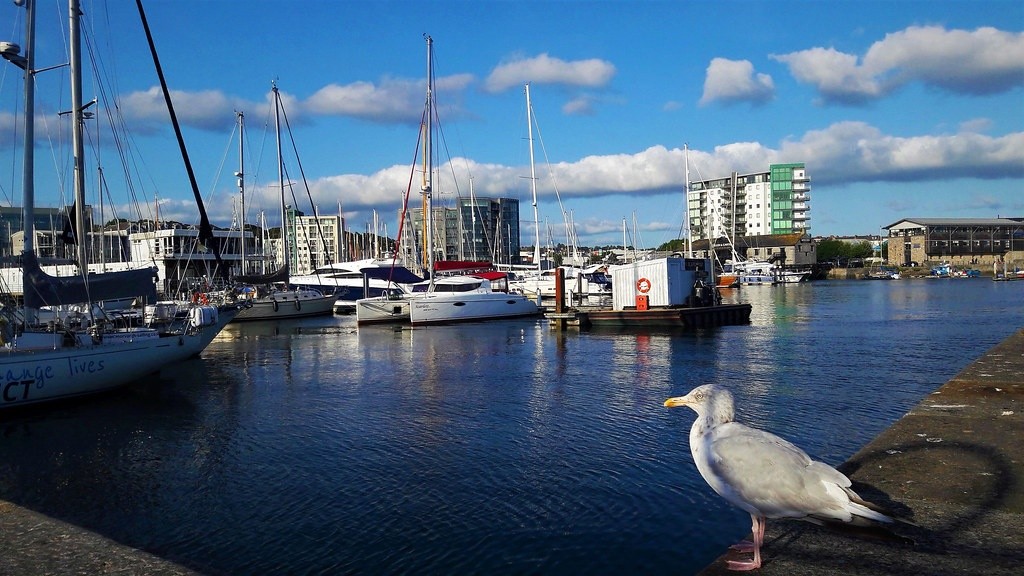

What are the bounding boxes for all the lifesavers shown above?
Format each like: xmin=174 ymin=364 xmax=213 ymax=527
xmin=637 ymin=278 xmax=651 ymax=293
xmin=64 ymin=311 xmax=87 ymax=330
xmin=193 ymin=293 xmax=207 ymax=305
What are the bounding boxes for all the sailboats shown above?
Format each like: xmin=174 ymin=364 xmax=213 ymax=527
xmin=1 ymin=0 xmax=838 ymax=413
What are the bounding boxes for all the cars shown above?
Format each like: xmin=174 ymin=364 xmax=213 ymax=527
xmin=902 ymin=261 xmax=918 ymax=267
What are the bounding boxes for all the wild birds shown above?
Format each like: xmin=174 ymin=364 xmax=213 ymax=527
xmin=661 ymin=384 xmax=919 ymax=571
